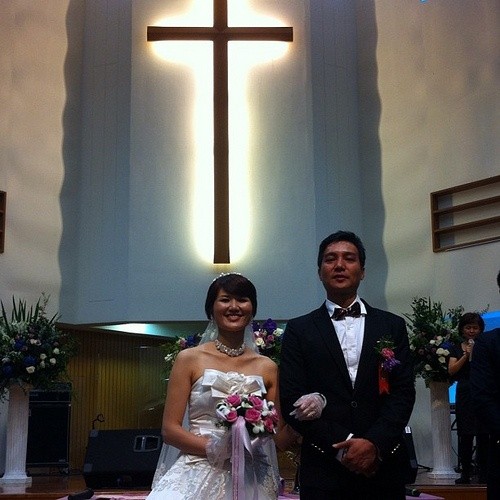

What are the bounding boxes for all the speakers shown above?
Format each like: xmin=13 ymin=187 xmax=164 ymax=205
xmin=82 ymin=424 xmax=163 ymax=489
xmin=25 ymin=383 xmax=71 ymax=478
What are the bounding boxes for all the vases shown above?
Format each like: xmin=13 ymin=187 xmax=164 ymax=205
xmin=0 ymin=383 xmax=32 ymax=496
xmin=427 ymin=382 xmax=461 ymax=485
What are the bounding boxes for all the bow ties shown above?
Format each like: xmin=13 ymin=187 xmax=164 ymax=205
xmin=331 ymin=302 xmax=361 ymax=321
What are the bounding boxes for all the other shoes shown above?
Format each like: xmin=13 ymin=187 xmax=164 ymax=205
xmin=455 ymin=477 xmax=471 ymax=484
xmin=471 ymin=476 xmax=485 ymax=484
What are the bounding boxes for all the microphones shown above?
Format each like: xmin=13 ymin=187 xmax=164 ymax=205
xmin=466 ymin=338 xmax=476 ymax=362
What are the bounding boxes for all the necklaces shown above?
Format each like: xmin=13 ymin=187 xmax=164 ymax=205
xmin=214 ymin=338 xmax=246 ymax=357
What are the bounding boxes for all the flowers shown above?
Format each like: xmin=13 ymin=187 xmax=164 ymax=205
xmin=159 ymin=331 xmax=204 ymax=378
xmin=215 ymin=394 xmax=279 ymax=438
xmin=0 ymin=294 xmax=77 ymax=400
xmin=403 ymin=297 xmax=490 ymax=390
xmin=252 ymin=319 xmax=285 ymax=366
xmin=374 ymin=336 xmax=402 ymax=370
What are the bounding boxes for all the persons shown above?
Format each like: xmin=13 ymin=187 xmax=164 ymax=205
xmin=449 ymin=271 xmax=500 ymax=500
xmin=145 ymin=272 xmax=326 ymax=500
xmin=278 ymin=231 xmax=417 ymax=500
